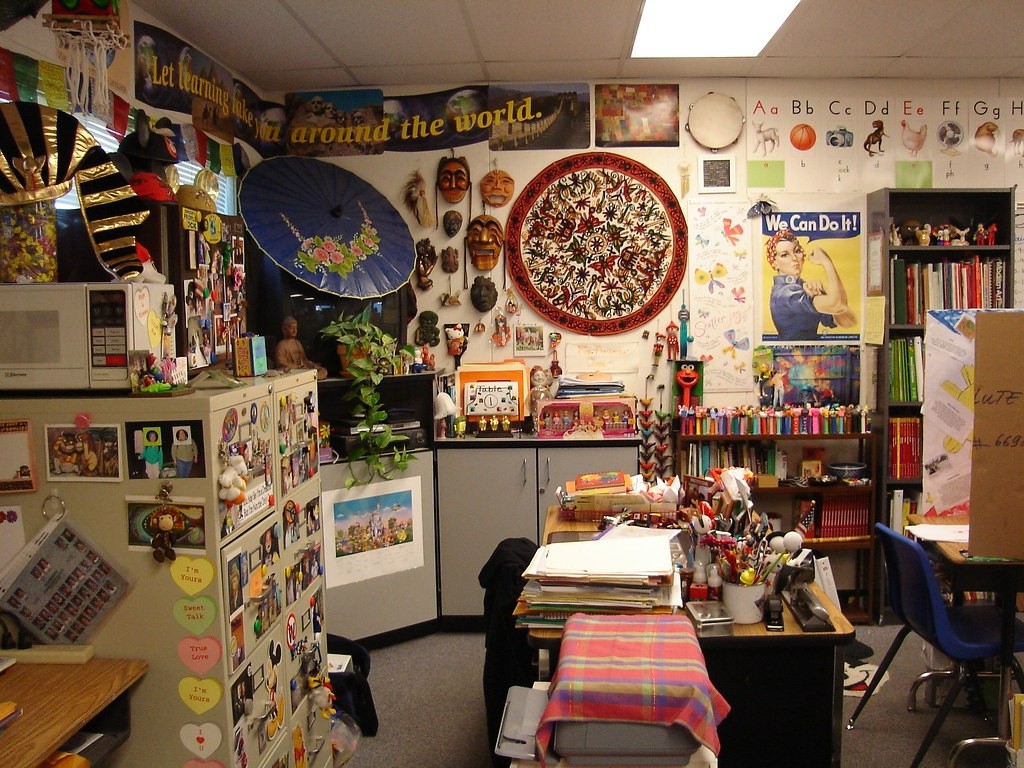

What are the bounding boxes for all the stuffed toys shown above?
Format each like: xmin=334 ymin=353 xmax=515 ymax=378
xmin=149 ymin=506 xmax=186 ymax=563
xmin=218 ymin=455 xmax=248 ymax=504
xmin=445 ymin=323 xmax=468 ymax=355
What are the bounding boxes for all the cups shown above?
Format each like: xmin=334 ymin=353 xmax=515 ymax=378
xmin=722 ymin=581 xmax=766 ymax=624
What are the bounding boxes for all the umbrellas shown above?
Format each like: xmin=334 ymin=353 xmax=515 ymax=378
xmin=238 ymin=154 xmax=419 ymax=301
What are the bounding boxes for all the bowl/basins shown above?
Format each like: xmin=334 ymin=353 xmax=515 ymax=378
xmin=828 ymin=462 xmax=867 ymax=482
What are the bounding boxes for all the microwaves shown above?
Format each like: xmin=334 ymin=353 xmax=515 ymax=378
xmin=0 ymin=283 xmax=176 ymax=389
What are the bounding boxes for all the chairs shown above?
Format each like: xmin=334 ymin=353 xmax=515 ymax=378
xmin=845 ymin=524 xmax=1024 ymax=768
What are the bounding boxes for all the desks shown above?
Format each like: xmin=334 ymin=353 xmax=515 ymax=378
xmin=526 ymin=505 xmax=853 ymax=768
xmin=0 ymin=656 xmax=148 ymax=768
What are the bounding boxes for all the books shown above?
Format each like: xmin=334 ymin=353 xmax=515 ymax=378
xmin=512 ymin=535 xmax=683 ymax=629
xmin=1008 ymin=693 xmax=1024 ymax=752
xmin=888 ymin=490 xmax=923 ymax=542
xmin=680 ymin=440 xmax=787 ymax=487
xmin=888 ymin=336 xmax=925 ymax=403
xmin=565 ymin=468 xmax=633 ymax=496
xmin=793 ymin=493 xmax=870 ymax=538
xmin=889 ymin=254 xmax=1011 ymax=325
xmin=888 ymin=418 xmax=923 ymax=481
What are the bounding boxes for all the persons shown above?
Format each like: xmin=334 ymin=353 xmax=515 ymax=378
xmin=6 ymin=529 xmax=119 ymax=644
xmin=276 ymin=316 xmax=328 ymax=380
xmin=138 ymin=431 xmax=163 ymax=478
xmin=171 ymin=429 xmax=198 ymax=478
xmin=528 ymin=364 xmax=554 ymax=437
xmin=479 ymin=414 xmax=510 ymax=432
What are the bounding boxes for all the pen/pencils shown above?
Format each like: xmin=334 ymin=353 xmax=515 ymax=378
xmin=0 ymin=708 xmax=23 ymax=731
xmin=965 ymin=556 xmax=1018 ymax=563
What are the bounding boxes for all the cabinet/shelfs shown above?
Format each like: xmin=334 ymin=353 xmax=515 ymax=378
xmin=866 ymin=185 xmax=1015 ymax=624
xmin=434 ymin=446 xmax=641 ymax=625
xmin=670 ymin=416 xmax=876 ymax=626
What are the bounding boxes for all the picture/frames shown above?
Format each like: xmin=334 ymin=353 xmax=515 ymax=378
xmin=513 ymin=323 xmax=547 ymax=357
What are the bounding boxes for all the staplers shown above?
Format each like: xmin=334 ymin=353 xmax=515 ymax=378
xmin=766 ymin=594 xmax=785 ymax=632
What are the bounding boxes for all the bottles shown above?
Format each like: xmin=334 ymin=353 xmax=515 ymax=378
xmin=693 ymin=562 xmax=707 ymax=583
xmin=707 ymin=565 xmax=723 ymax=601
